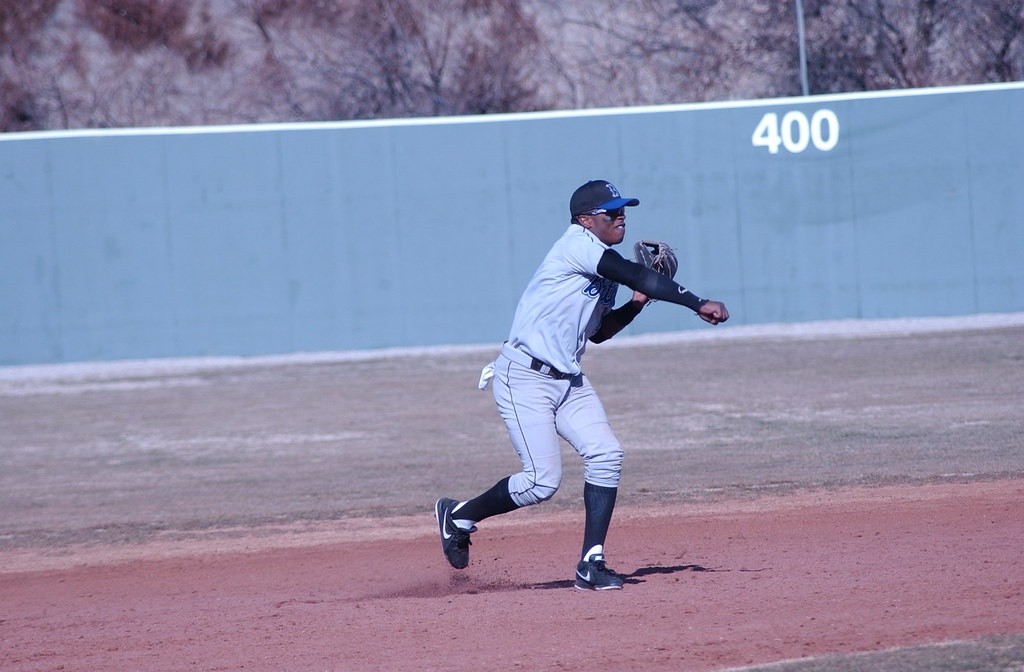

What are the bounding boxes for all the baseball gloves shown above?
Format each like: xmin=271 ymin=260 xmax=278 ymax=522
xmin=630 ymin=240 xmax=679 ymax=283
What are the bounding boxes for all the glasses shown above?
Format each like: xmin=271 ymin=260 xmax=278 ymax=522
xmin=574 ymin=206 xmax=625 ymax=219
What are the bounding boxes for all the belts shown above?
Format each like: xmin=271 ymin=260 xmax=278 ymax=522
xmin=530 ymin=358 xmax=573 ymax=380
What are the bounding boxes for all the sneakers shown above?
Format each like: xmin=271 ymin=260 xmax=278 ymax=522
xmin=572 ymin=553 xmax=624 ymax=592
xmin=434 ymin=497 xmax=477 ymax=570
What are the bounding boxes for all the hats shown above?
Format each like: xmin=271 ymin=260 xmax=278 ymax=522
xmin=568 ymin=178 xmax=640 ymax=211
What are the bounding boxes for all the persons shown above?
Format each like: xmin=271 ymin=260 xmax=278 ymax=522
xmin=427 ymin=177 xmax=732 ymax=595
